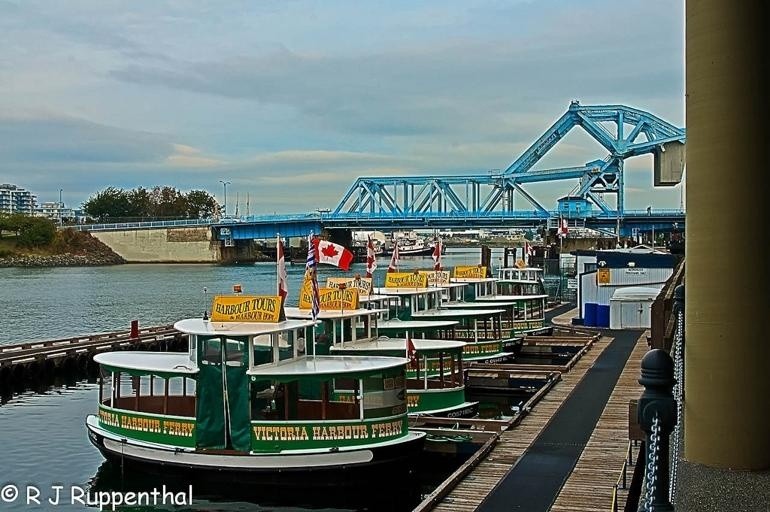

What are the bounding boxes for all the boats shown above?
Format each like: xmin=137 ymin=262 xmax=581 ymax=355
xmin=387 ymin=238 xmax=436 ymax=256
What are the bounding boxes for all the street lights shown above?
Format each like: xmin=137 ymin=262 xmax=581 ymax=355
xmin=57 ymin=189 xmax=63 ymax=218
xmin=220 ymin=181 xmax=231 ymax=217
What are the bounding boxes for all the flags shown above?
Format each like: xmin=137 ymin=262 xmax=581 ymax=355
xmin=525 ymin=241 xmax=533 ymax=256
xmin=387 ymin=242 xmax=398 ymax=272
xmin=314 ymin=238 xmax=354 ymax=271
xmin=367 ymin=234 xmax=378 ymax=277
xmin=561 ymin=214 xmax=569 ymax=234
xmin=305 ymin=237 xmax=321 ymax=321
xmin=431 ymin=243 xmax=441 ymax=271
xmin=277 ymin=239 xmax=289 ymax=305
xmin=405 ymin=335 xmax=419 ymax=369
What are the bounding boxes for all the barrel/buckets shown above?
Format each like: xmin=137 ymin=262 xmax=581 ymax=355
xmin=597 ymin=304 xmax=609 ymax=328
xmin=584 ymin=303 xmax=597 ymax=327
